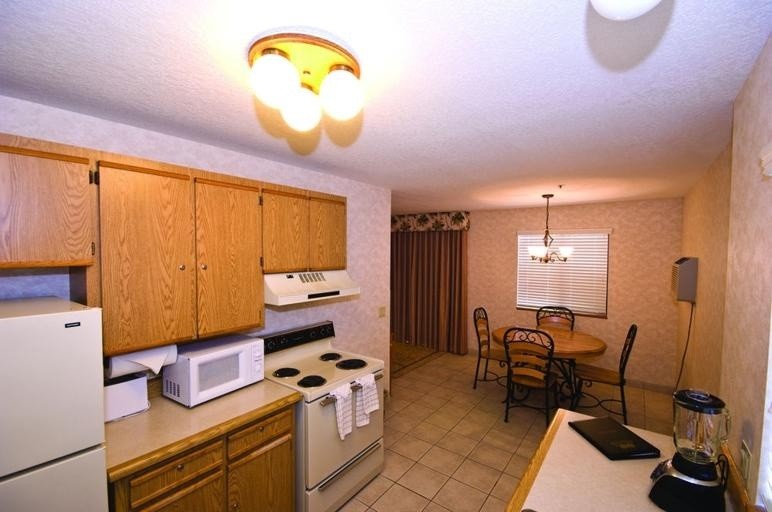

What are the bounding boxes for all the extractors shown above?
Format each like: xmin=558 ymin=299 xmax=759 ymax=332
xmin=263 ymin=271 xmax=361 ymax=307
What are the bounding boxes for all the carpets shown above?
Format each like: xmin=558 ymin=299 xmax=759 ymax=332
xmin=391 ymin=339 xmax=444 ymax=377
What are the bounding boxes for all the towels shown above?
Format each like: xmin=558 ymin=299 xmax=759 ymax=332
xmin=329 ymin=383 xmax=352 ymax=442
xmin=355 ymin=373 xmax=379 ymax=428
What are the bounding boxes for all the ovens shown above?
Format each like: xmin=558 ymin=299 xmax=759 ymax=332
xmin=267 ymin=370 xmax=385 ymax=512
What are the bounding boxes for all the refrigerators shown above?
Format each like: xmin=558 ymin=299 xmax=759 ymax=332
xmin=0 ymin=297 xmax=110 ymax=512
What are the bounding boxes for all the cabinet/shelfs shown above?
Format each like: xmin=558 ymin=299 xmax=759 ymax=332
xmin=110 ymin=392 xmax=303 ymax=511
xmin=97 ymin=160 xmax=264 ymax=358
xmin=260 ymin=188 xmax=348 ymax=274
xmin=0 ymin=146 xmax=94 ymax=269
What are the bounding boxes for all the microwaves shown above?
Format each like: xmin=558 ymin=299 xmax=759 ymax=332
xmin=161 ymin=334 xmax=266 ymax=409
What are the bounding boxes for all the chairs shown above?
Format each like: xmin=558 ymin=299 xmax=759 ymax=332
xmin=536 ymin=306 xmax=575 ymax=332
xmin=502 ymin=326 xmax=556 ymax=429
xmin=473 ymin=306 xmax=511 ymax=390
xmin=572 ymin=324 xmax=638 ymax=425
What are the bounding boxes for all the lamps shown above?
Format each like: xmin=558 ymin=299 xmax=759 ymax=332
xmin=527 ymin=192 xmax=573 ymax=266
xmin=246 ymin=34 xmax=366 ymax=132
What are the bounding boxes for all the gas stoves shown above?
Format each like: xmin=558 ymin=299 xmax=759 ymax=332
xmin=256 ymin=320 xmax=386 ymax=403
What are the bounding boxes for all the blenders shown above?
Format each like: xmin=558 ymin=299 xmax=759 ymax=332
xmin=649 ymin=389 xmax=732 ymax=512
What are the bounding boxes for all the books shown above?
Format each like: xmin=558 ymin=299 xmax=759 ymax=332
xmin=568 ymin=415 xmax=660 ymax=460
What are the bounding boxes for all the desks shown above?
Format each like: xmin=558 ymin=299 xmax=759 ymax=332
xmin=506 ymin=408 xmax=739 ymax=512
xmin=492 ymin=323 xmax=608 ymax=404
